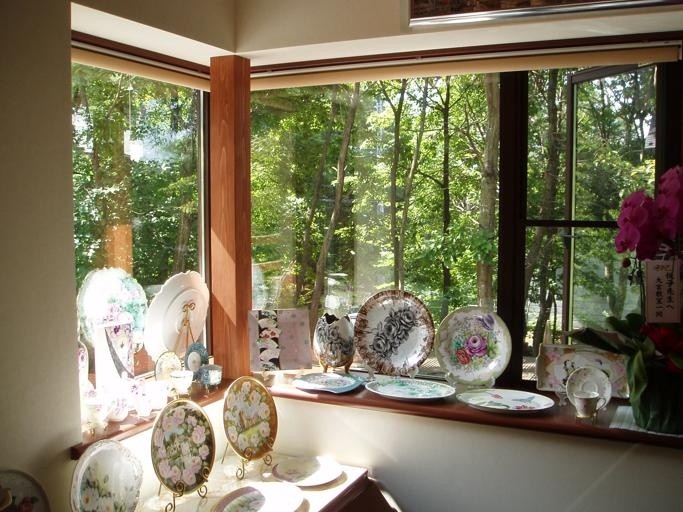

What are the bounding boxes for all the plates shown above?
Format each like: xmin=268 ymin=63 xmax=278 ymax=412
xmin=433 ymin=305 xmax=515 ymax=385
xmin=152 ymin=350 xmax=184 ymax=381
xmin=1 ymin=466 xmax=51 ymax=512
xmin=214 ymin=451 xmax=342 ymax=512
xmin=183 ymin=342 xmax=209 ymax=370
xmin=65 ymin=438 xmax=143 ymax=512
xmin=291 ymin=372 xmax=359 ymax=394
xmin=455 ymin=389 xmax=556 ymax=417
xmin=530 ymin=341 xmax=632 ymax=402
xmin=219 ymin=375 xmax=280 ymax=460
xmin=352 ymin=288 xmax=433 ymax=375
xmin=561 ymin=364 xmax=613 ymax=411
xmin=363 ymin=375 xmax=456 ymax=405
xmin=75 ymin=265 xmax=209 ymax=366
xmin=149 ymin=402 xmax=216 ymax=497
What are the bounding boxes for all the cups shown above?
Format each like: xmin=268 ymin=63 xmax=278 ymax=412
xmin=570 ymin=390 xmax=608 ymax=420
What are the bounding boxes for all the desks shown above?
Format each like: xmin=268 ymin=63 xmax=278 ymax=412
xmin=120 ymin=450 xmax=402 ymax=512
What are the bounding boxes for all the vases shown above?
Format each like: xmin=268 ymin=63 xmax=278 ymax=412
xmin=622 ymin=354 xmax=682 ymax=438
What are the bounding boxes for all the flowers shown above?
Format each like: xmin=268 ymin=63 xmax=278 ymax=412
xmin=565 ymin=165 xmax=682 ymax=364
